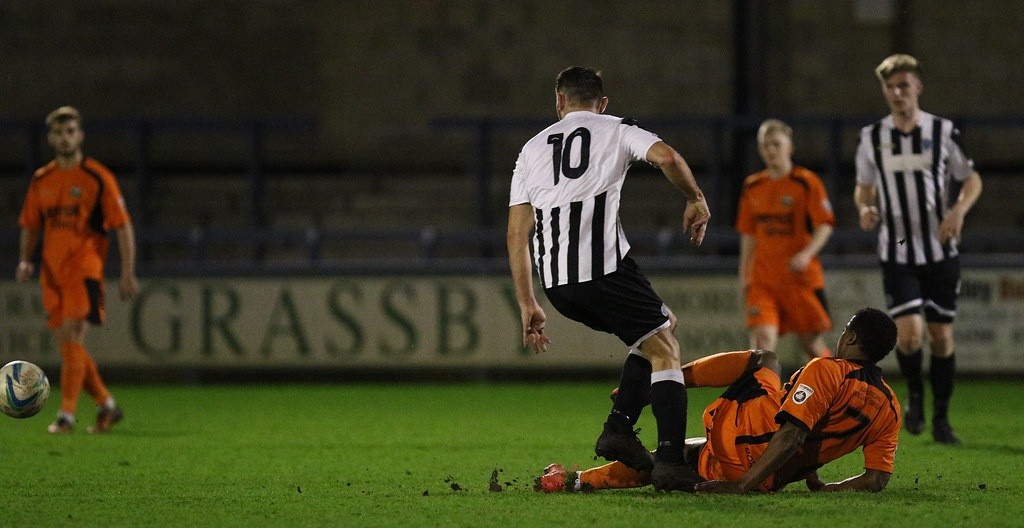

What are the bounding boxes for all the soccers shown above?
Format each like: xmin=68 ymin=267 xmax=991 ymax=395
xmin=0 ymin=360 xmax=51 ymax=418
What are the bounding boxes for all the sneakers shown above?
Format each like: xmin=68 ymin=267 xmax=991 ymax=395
xmin=651 ymin=459 xmax=699 ymax=492
xmin=932 ymin=427 xmax=961 ymax=448
xmin=88 ymin=406 xmax=124 ymax=433
xmin=611 ymin=389 xmax=619 ymax=401
xmin=49 ymin=414 xmax=76 ymax=434
xmin=595 ymin=420 xmax=655 ymax=472
xmin=539 ymin=463 xmax=568 ymax=492
xmin=905 ymin=391 xmax=926 ymax=435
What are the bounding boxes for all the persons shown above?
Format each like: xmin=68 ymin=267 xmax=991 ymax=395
xmin=535 ymin=309 xmax=902 ymax=493
xmin=15 ymin=107 xmax=140 ymax=432
xmin=854 ymin=53 xmax=984 ymax=441
xmin=508 ymin=66 xmax=712 ymax=494
xmin=739 ymin=120 xmax=835 ymax=358
